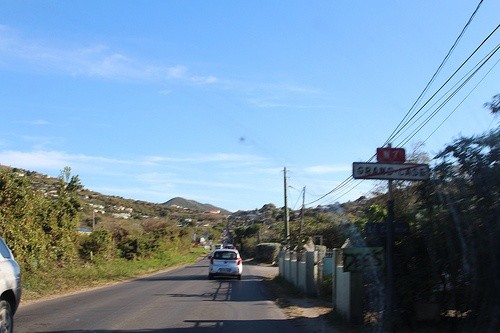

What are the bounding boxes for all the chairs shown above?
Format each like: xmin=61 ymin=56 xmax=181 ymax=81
xmin=229 ymin=254 xmax=234 ymax=259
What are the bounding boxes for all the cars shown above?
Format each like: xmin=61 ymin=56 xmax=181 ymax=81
xmin=208 ymin=249 xmax=243 ymax=280
xmin=0 ymin=235 xmax=23 ymax=333
